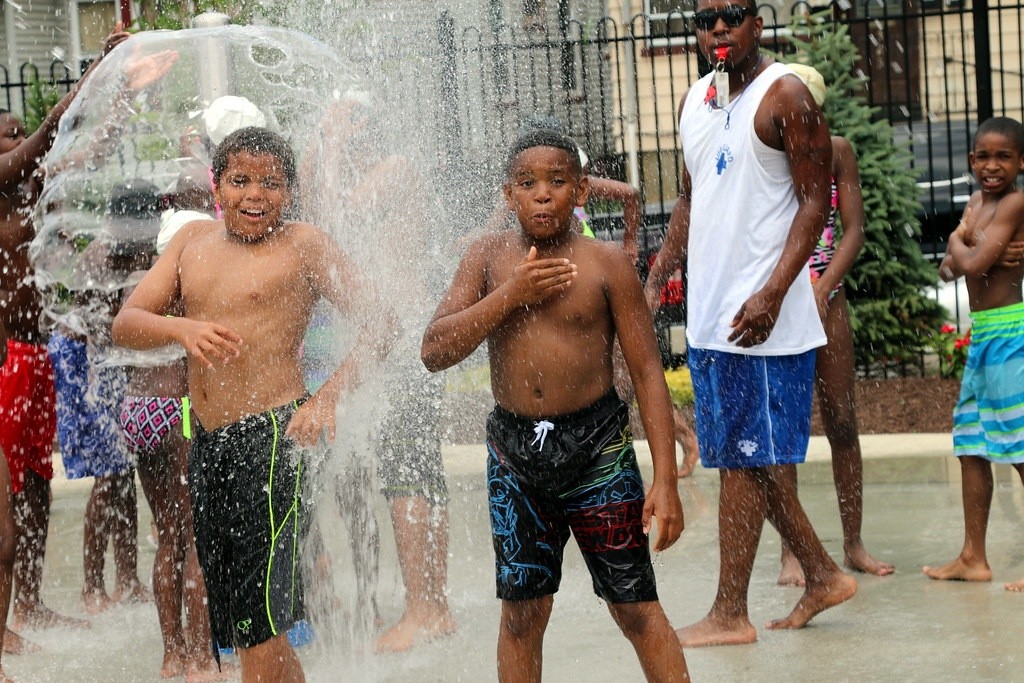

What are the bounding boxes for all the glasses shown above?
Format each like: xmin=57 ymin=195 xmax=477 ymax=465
xmin=692 ymin=3 xmax=758 ymax=31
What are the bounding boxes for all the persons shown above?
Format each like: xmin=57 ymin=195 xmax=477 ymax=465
xmin=0 ymin=21 xmax=454 ymax=683
xmin=644 ymin=0 xmax=857 ymax=646
xmin=110 ymin=127 xmax=405 ymax=683
xmin=920 ymin=117 xmax=1024 ymax=592
xmin=421 ymin=130 xmax=699 ymax=683
xmin=777 ymin=63 xmax=897 ymax=587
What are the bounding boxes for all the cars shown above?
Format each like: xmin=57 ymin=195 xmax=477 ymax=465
xmin=583 ymin=198 xmax=689 ymax=372
xmin=853 ymin=119 xmax=983 ymax=279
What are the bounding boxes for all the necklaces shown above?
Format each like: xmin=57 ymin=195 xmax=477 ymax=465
xmin=708 ymin=56 xmax=761 ymax=129
